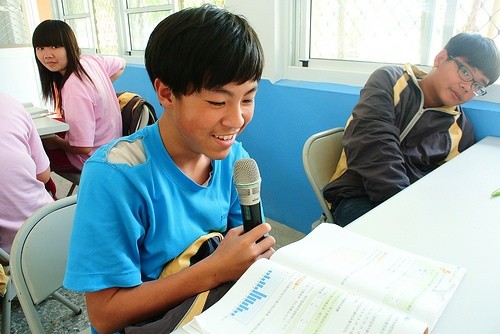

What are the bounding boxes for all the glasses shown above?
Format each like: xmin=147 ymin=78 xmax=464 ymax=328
xmin=449 ymin=55 xmax=487 ymax=96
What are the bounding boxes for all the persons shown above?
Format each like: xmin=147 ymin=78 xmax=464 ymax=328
xmin=62 ymin=4 xmax=276 ymax=334
xmin=33 ymin=19 xmax=128 ymax=198
xmin=0 ymin=87 xmax=58 ymax=267
xmin=322 ymin=32 xmax=500 ymax=227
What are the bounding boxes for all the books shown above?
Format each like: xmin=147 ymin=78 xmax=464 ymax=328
xmin=170 ymin=223 xmax=466 ymax=334
xmin=22 ymin=102 xmax=49 ymax=115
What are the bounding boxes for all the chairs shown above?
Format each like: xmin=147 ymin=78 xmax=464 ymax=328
xmin=54 ymin=92 xmax=150 ymax=197
xmin=301 ymin=127 xmax=345 ymax=231
xmin=9 ymin=193 xmax=91 ymax=334
xmin=0 ymin=244 xmax=82 ymax=334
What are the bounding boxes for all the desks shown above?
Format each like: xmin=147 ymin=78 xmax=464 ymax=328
xmin=342 ymin=132 xmax=499 ymax=334
xmin=33 ymin=117 xmax=70 ymax=136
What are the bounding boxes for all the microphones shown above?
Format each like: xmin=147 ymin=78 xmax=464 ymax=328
xmin=234 ymin=159 xmax=265 ymax=244
